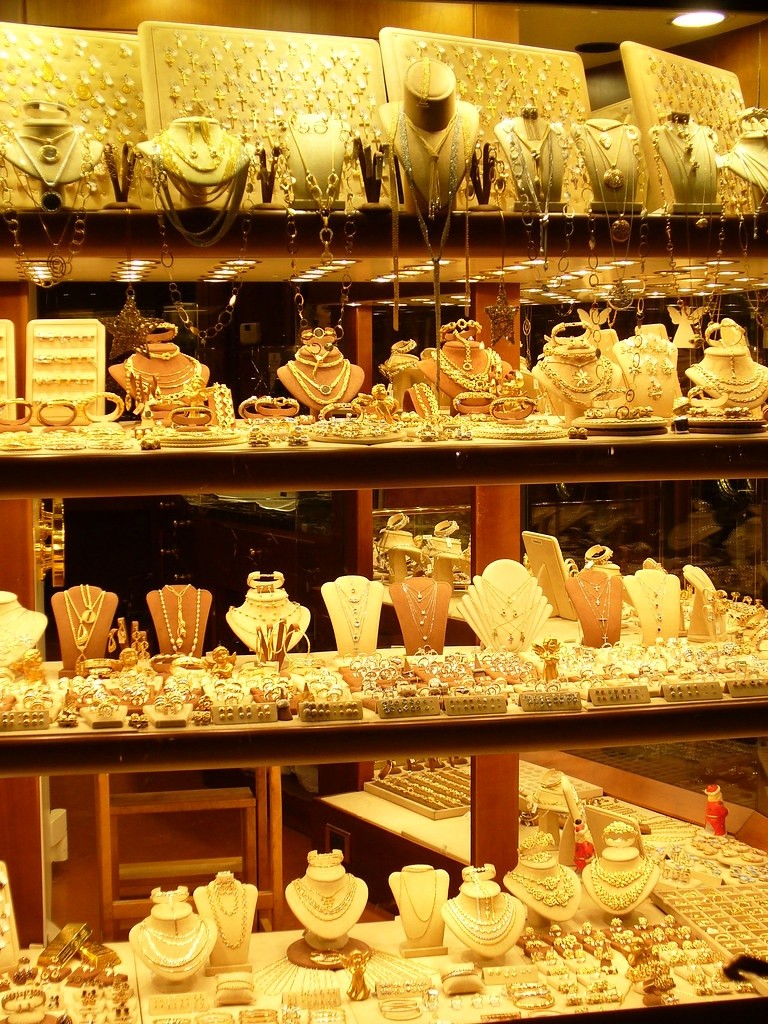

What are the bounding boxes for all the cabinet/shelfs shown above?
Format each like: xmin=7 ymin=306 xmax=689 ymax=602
xmin=0 ymin=212 xmax=767 ymax=1024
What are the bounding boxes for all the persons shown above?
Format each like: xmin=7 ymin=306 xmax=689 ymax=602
xmin=704 ymin=784 xmax=728 ymax=837
xmin=573 ymin=823 xmax=595 ymax=871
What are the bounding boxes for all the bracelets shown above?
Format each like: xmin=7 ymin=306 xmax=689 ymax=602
xmin=1 ymin=989 xmax=46 ymax=1013
xmin=379 ymin=998 xmax=423 ymax=1021
xmin=540 ymin=768 xmax=562 ymax=790
xmin=435 ymin=520 xmax=457 ymax=538
xmin=173 ymin=406 xmax=212 ymax=427
xmin=585 ymin=545 xmax=613 ymax=564
xmin=387 ymin=513 xmax=407 ymax=531
xmin=152 ymin=978 xmax=346 ymax=1024
xmin=509 ymin=982 xmax=555 ymax=1010
xmin=0 ymin=390 xmax=125 ymax=427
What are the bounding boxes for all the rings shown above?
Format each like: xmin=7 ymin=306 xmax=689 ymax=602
xmin=241 ymin=408 xmax=750 ymax=447
xmin=0 ymin=592 xmax=768 ymax=716
xmin=451 ymin=992 xmax=500 ymax=1010
xmin=524 ymin=892 xmax=768 ymax=1014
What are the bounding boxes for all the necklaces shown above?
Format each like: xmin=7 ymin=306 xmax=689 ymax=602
xmin=136 ymin=831 xmax=572 ymax=995
xmin=124 ymin=343 xmax=203 ymax=402
xmin=691 ymin=362 xmax=768 ymax=403
xmin=289 ymin=348 xmax=613 ymax=413
xmin=0 ymin=574 xmax=668 ymax=663
xmin=0 ymin=101 xmax=768 ymax=355
